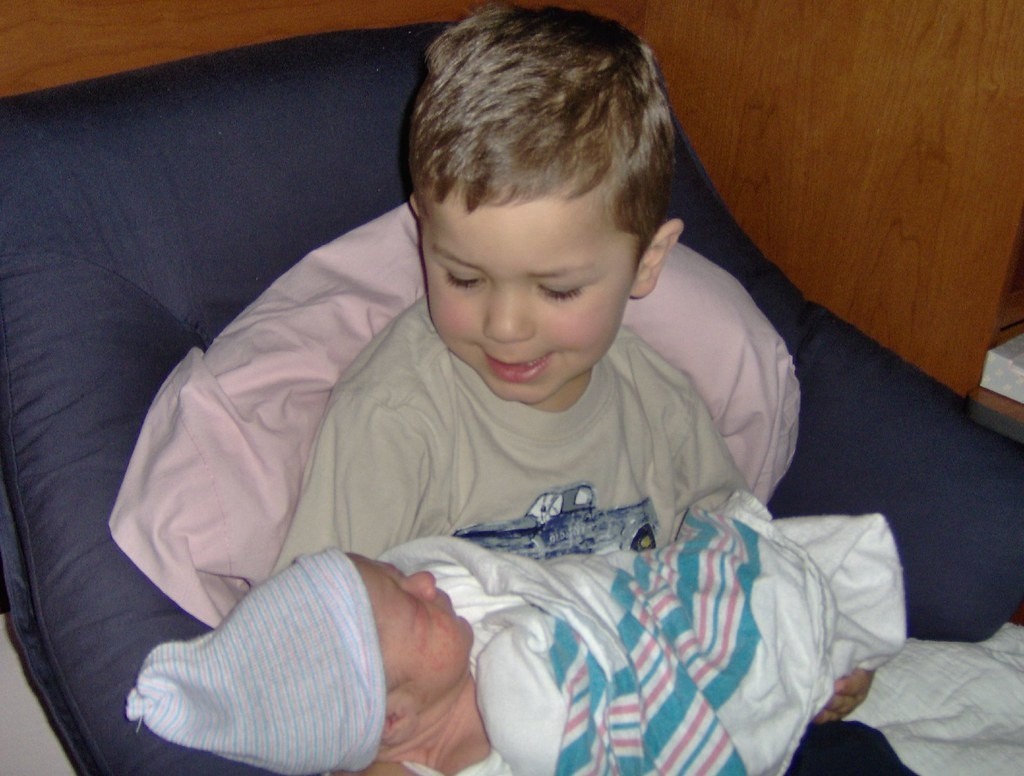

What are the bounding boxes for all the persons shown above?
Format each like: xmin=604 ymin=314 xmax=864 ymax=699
xmin=124 ymin=489 xmax=906 ymax=776
xmin=271 ymin=7 xmax=918 ymax=776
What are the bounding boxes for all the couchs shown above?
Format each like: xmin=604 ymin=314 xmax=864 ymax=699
xmin=0 ymin=22 xmax=1024 ymax=776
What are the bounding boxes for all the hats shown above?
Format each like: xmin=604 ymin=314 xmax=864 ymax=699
xmin=126 ymin=548 xmax=386 ymax=776
